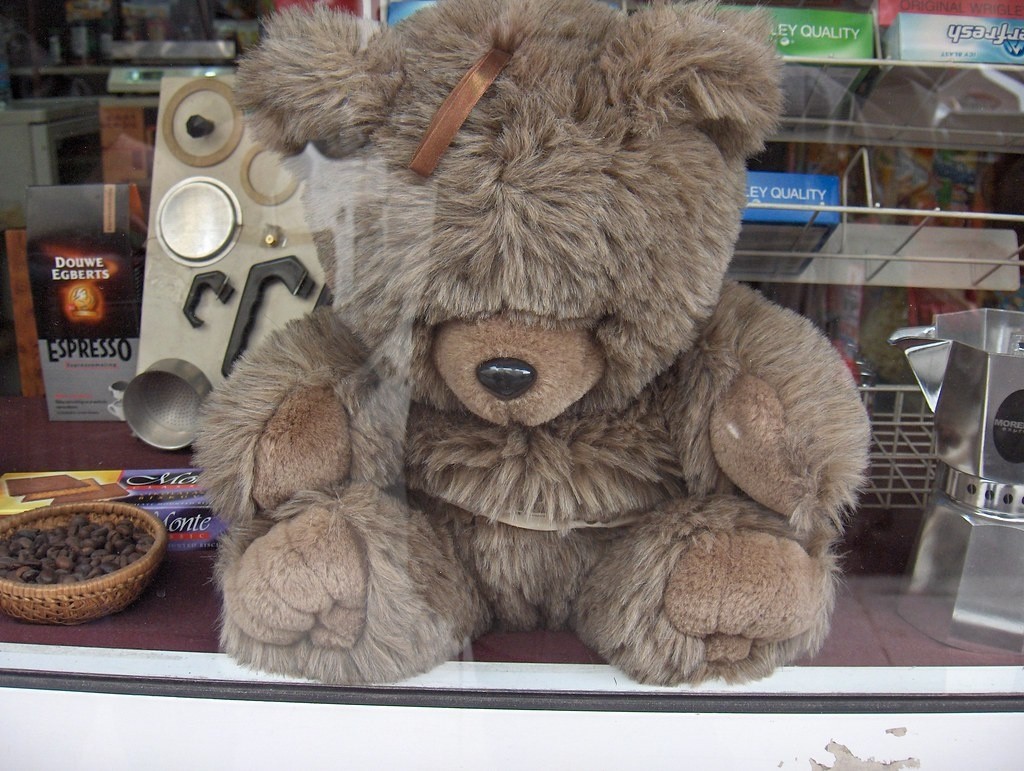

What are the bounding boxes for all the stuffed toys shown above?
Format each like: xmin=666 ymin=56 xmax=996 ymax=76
xmin=193 ymin=1 xmax=870 ymax=686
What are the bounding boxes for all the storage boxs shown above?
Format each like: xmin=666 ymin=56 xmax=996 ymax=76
xmin=854 ymin=12 xmax=1024 ymax=148
xmin=0 ymin=468 xmax=232 ymax=552
xmin=867 ymin=0 xmax=1024 ymax=39
xmin=29 ymin=183 xmax=147 ymax=419
xmin=709 ymin=6 xmax=874 ymax=131
xmin=727 ymin=171 xmax=843 ymax=276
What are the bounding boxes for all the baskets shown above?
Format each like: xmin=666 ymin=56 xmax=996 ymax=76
xmin=1 ymin=500 xmax=170 ymax=625
